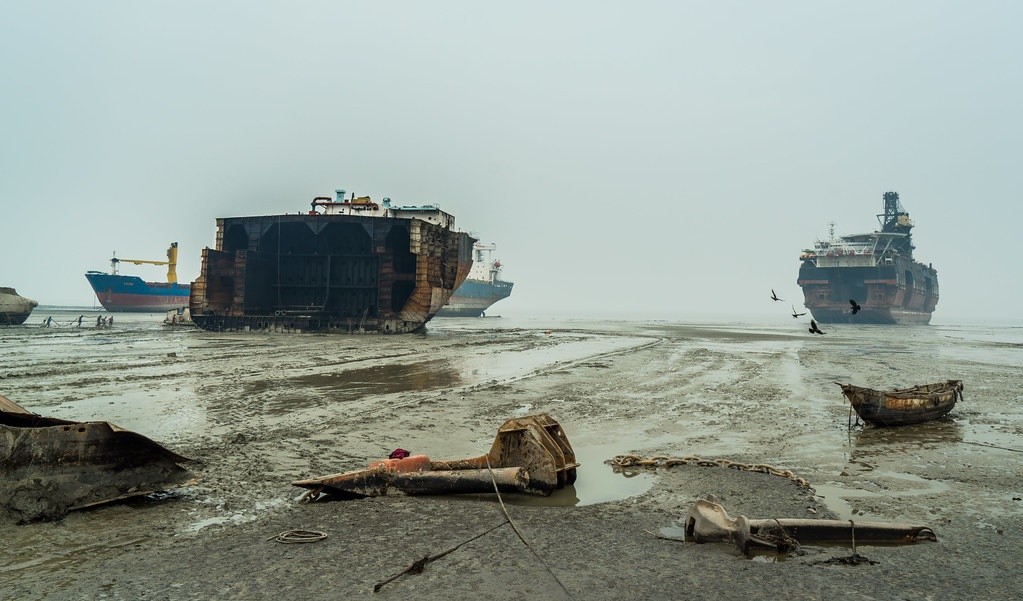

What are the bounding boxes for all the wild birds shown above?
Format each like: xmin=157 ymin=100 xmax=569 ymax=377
xmin=809 ymin=319 xmax=827 ymax=335
xmin=770 ymin=289 xmax=786 ymax=302
xmin=849 ymin=299 xmax=861 ymax=315
xmin=792 ymin=304 xmax=807 ymax=318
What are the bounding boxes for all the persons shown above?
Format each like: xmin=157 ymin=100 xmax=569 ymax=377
xmin=96 ymin=315 xmax=113 ymax=328
xmin=47 ymin=317 xmax=52 ymax=328
xmin=6 ymin=313 xmax=11 ymax=327
xmin=76 ymin=315 xmax=83 ymax=327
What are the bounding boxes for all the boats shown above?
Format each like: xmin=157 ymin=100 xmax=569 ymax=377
xmin=84 ymin=228 xmax=514 ymax=317
xmin=0 ymin=287 xmax=38 ymax=325
xmin=834 ymin=379 xmax=964 ymax=426
xmin=797 ymin=192 xmax=939 ymax=326
